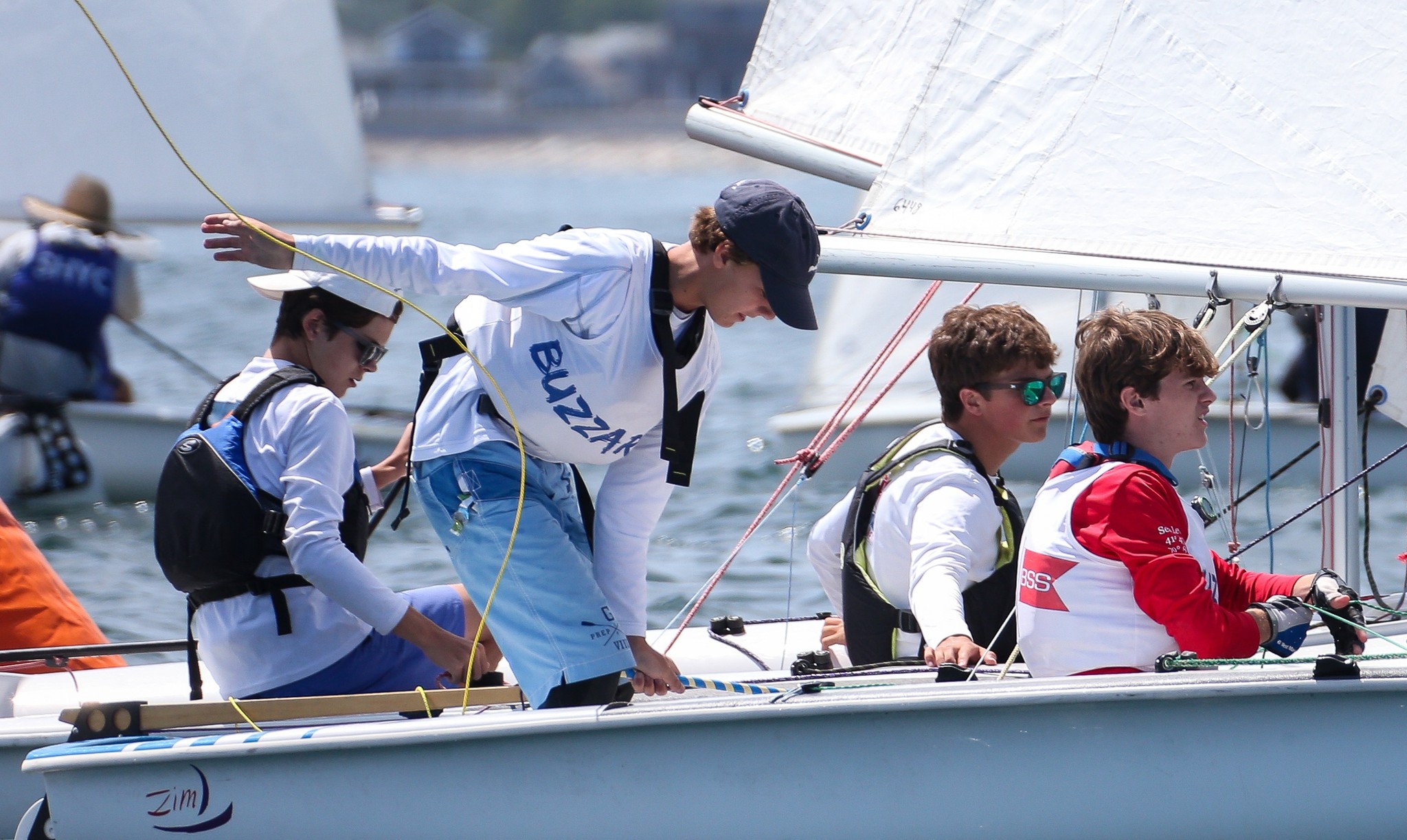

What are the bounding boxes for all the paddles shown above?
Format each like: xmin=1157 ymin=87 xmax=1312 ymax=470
xmin=58 ymin=684 xmax=529 ymax=741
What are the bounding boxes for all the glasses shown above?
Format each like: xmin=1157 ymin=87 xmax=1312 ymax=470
xmin=989 ymin=370 xmax=1069 ymax=408
xmin=325 ymin=319 xmax=388 ymax=371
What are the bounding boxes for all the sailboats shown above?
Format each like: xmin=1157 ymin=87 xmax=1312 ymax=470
xmin=0 ymin=0 xmax=1407 ymax=838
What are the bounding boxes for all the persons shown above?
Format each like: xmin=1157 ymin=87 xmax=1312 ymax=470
xmin=0 ymin=178 xmax=141 ymax=406
xmin=198 ymin=180 xmax=821 ymax=714
xmin=1010 ymin=306 xmax=1369 ymax=680
xmin=805 ymin=302 xmax=1066 ymax=669
xmin=155 ymin=268 xmax=501 ymax=701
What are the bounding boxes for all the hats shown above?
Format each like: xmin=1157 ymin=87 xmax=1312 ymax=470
xmin=247 ymin=270 xmax=401 ymax=316
xmin=715 ymin=179 xmax=823 ymax=331
